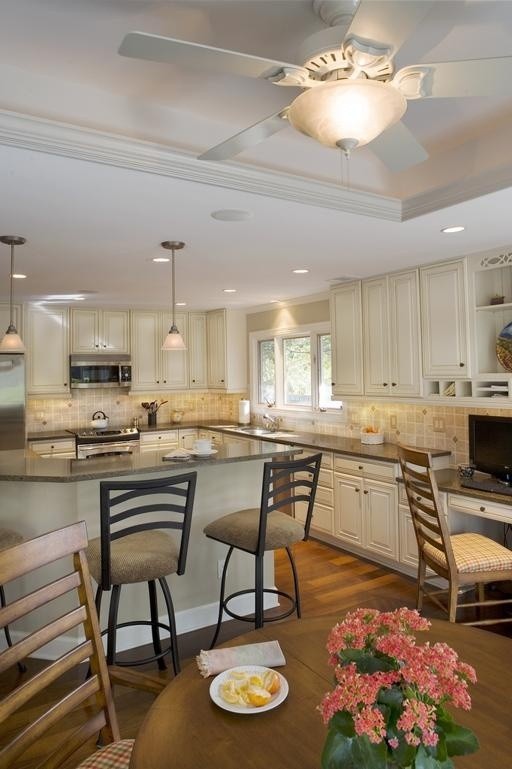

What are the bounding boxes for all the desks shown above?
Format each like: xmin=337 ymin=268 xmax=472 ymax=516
xmin=396 ymin=468 xmax=511 ymax=527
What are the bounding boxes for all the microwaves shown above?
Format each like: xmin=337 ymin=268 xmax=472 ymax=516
xmin=70 ymin=361 xmax=132 ymax=388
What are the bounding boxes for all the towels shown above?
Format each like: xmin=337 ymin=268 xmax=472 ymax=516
xmin=195 ymin=639 xmax=287 ymax=677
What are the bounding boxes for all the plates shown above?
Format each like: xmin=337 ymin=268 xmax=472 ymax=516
xmin=209 ymin=664 xmax=290 ymax=715
xmin=495 ymin=321 xmax=512 ymax=373
xmin=190 ymin=449 xmax=217 ymax=458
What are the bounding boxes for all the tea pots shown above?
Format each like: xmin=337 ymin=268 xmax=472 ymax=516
xmin=91 ymin=412 xmax=109 ymax=429
xmin=171 ymin=409 xmax=184 ymax=423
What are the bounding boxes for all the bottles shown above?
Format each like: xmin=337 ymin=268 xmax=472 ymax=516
xmin=132 ymin=417 xmax=139 ymax=427
xmin=239 ymin=398 xmax=251 ymax=425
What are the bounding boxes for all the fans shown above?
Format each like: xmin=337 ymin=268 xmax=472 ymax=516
xmin=117 ymin=1 xmax=512 ymax=161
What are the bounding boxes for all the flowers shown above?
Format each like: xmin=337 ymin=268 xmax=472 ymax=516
xmin=310 ymin=605 xmax=478 ymax=769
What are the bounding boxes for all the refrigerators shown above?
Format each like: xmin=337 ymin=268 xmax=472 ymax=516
xmin=0 ymin=353 xmax=27 ymax=451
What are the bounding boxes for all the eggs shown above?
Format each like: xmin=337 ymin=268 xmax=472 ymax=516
xmin=361 ymin=426 xmax=379 ymax=435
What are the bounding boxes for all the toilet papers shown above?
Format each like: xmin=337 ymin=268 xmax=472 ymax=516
xmin=239 ymin=400 xmax=250 ymax=423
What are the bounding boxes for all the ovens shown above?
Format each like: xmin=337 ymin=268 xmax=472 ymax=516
xmin=77 ymin=435 xmax=139 ymax=459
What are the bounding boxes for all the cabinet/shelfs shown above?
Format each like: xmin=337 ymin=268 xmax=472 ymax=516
xmin=329 ymin=246 xmax=512 ymax=409
xmin=29 ymin=438 xmax=77 ymax=461
xmin=0 ymin=303 xmax=22 ymax=357
xmin=139 ymin=427 xmax=290 ymax=454
xmin=290 ymin=445 xmax=451 ymax=590
xmin=24 ymin=306 xmax=230 ymax=394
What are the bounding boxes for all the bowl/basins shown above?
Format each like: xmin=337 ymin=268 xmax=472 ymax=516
xmin=361 ymin=433 xmax=384 ymax=444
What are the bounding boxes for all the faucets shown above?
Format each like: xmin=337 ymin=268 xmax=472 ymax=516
xmin=263 ymin=414 xmax=276 ymax=424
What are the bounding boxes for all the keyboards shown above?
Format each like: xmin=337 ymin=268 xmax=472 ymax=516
xmin=461 ymin=480 xmax=512 ymax=496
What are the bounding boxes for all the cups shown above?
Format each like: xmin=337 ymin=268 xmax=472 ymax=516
xmin=193 ymin=440 xmax=212 ymax=452
xmin=455 ymin=462 xmax=475 ymax=479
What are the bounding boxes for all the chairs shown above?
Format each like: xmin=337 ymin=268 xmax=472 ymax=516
xmin=83 ymin=471 xmax=199 ymax=676
xmin=204 ymin=452 xmax=323 ymax=656
xmin=397 ymin=444 xmax=511 ymax=627
xmin=0 ymin=526 xmax=29 ymax=671
xmin=0 ymin=521 xmax=134 ymax=767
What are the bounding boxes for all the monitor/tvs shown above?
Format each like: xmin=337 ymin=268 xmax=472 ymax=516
xmin=468 ymin=414 xmax=512 ymax=485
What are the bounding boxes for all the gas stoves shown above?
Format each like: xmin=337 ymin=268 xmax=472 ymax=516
xmin=67 ymin=425 xmax=139 ymax=434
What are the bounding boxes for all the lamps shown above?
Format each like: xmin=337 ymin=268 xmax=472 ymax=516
xmin=287 ymin=80 xmax=408 ymax=160
xmin=1 ymin=234 xmax=27 ymax=356
xmin=161 ymin=240 xmax=189 ymax=351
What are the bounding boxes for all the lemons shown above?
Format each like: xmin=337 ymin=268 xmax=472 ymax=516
xmin=219 ymin=670 xmax=281 ymax=707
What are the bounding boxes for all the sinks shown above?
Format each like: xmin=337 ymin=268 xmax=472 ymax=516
xmin=234 ymin=425 xmax=293 ymax=435
